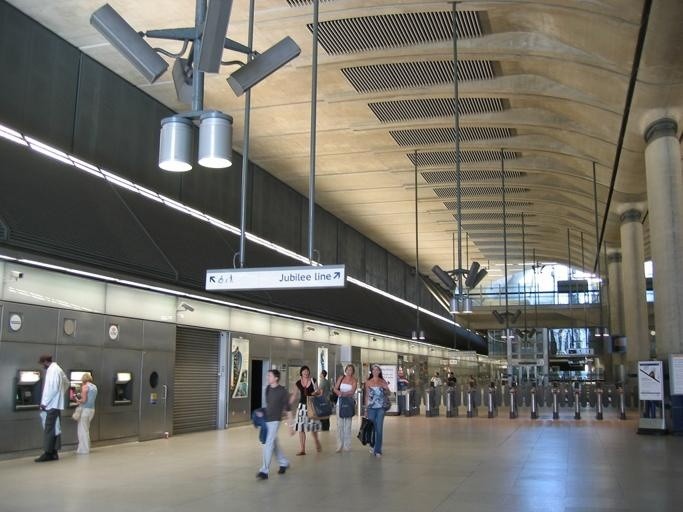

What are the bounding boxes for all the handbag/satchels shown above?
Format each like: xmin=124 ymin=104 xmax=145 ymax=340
xmin=357 ymin=416 xmax=375 ymax=448
xmin=338 ymin=397 xmax=356 ymax=418
xmin=307 ymin=393 xmax=330 ymax=420
xmin=313 ymin=395 xmax=332 ymax=417
xmin=333 ymin=375 xmax=345 ymax=405
xmin=382 ymin=393 xmax=391 ymax=411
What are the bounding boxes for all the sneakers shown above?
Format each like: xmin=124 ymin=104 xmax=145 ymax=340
xmin=335 ymin=448 xmax=342 ymax=453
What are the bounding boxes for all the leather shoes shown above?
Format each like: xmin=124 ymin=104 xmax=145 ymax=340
xmin=316 ymin=440 xmax=321 ymax=453
xmin=369 ymin=446 xmax=375 ymax=454
xmin=255 ymin=472 xmax=268 ymax=479
xmin=278 ymin=466 xmax=286 ymax=473
xmin=296 ymin=452 xmax=305 ymax=455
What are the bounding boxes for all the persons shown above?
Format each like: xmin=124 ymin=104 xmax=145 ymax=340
xmin=71 ymin=374 xmax=99 ymax=454
xmin=431 ymin=372 xmax=441 ymax=386
xmin=251 ymin=370 xmax=291 ymax=479
xmin=288 ymin=365 xmax=323 ymax=456
xmin=447 ymin=372 xmax=456 ymax=385
xmin=33 ymin=355 xmax=69 ymax=464
xmin=397 ymin=366 xmax=410 ymax=389
xmin=316 ymin=369 xmax=333 ymax=432
xmin=332 ymin=365 xmax=358 ymax=454
xmin=363 ymin=363 xmax=391 ymax=457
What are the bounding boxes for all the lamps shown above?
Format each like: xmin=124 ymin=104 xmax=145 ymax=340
xmin=90 ymin=0 xmax=301 ymax=171
xmin=431 ymin=231 xmax=486 ymax=314
xmin=492 ymin=308 xmax=536 ymax=338
